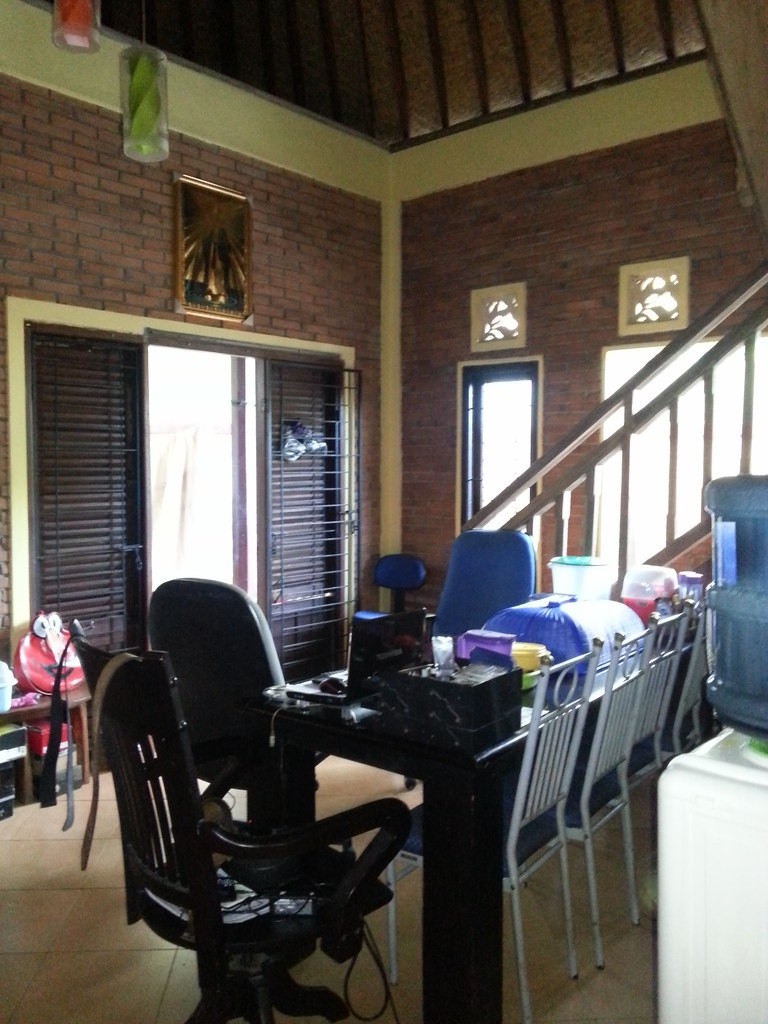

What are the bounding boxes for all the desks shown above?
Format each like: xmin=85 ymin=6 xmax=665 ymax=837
xmin=0 ymin=682 xmax=93 ymax=806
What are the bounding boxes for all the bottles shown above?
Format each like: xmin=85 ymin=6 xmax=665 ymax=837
xmin=703 ymin=475 xmax=768 ymax=735
xmin=678 ymin=571 xmax=703 ymax=610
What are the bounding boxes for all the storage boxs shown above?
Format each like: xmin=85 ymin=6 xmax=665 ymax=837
xmin=620 ymin=563 xmax=679 ymax=623
xmin=546 ymin=555 xmax=616 ymax=602
xmin=29 ymin=744 xmax=77 ymax=776
xmin=0 ymin=763 xmax=15 ymax=821
xmin=398 ymin=663 xmax=521 ymax=730
xmin=27 ymin=718 xmax=73 ymax=757
xmin=0 ymin=723 xmax=27 ymax=764
xmin=33 ymin=765 xmax=82 ymax=802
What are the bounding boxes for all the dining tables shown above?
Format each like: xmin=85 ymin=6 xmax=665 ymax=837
xmin=238 ymin=636 xmax=708 ymax=1024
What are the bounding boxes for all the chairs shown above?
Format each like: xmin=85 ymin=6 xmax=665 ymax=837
xmin=616 ymin=599 xmax=691 ymax=792
xmin=504 ymin=614 xmax=659 ymax=970
xmin=350 ymin=552 xmax=428 ymax=627
xmin=378 ymin=639 xmax=607 ymax=1024
xmin=641 ymin=601 xmax=708 ymax=761
xmin=147 ymin=577 xmax=334 ymax=819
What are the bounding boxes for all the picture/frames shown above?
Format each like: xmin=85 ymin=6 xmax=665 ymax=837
xmin=171 ymin=171 xmax=254 ymax=327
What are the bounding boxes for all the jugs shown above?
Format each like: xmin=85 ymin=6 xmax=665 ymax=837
xmin=456 ymin=626 xmax=518 ymax=668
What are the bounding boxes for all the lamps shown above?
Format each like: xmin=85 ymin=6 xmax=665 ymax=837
xmin=119 ymin=0 xmax=169 ymax=162
xmin=53 ymin=0 xmax=102 ymax=53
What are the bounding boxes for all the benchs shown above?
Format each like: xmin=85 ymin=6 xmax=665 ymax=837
xmin=72 ymin=634 xmax=414 ymax=1024
